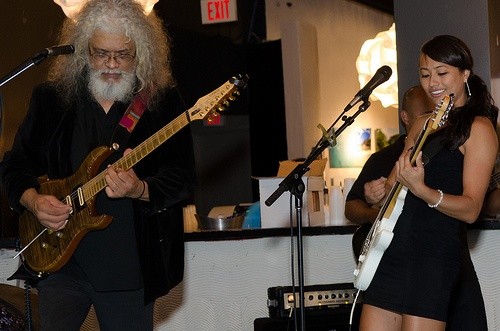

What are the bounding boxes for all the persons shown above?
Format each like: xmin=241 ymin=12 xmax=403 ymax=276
xmin=0 ymin=0 xmax=197 ymax=331
xmin=359 ymin=35 xmax=500 ymax=331
xmin=344 ymin=86 xmax=438 ymax=265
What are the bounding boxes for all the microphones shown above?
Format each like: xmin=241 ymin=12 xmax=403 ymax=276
xmin=345 ymin=66 xmax=392 ymax=112
xmin=40 ymin=44 xmax=75 ymax=55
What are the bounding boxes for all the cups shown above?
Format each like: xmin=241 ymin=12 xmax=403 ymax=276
xmin=330 ymin=185 xmax=345 ymax=226
xmin=329 ymin=144 xmax=343 ymax=167
xmin=342 ymin=178 xmax=355 ymax=198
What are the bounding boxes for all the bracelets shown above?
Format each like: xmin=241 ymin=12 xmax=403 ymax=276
xmin=136 ymin=181 xmax=145 ymax=199
xmin=428 ymin=189 xmax=444 ymax=208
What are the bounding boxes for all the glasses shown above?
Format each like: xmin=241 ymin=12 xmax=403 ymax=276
xmin=88 ymin=42 xmax=137 ymax=65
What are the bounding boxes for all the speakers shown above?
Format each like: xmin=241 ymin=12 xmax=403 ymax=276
xmin=286 ymin=309 xmax=362 ymax=331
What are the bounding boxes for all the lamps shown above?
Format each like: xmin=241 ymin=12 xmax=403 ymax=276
xmin=53 ymin=0 xmax=160 ymax=25
xmin=355 ymin=22 xmax=399 ymax=109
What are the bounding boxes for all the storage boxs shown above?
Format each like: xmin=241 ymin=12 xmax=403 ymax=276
xmin=251 ymin=157 xmax=329 ymax=228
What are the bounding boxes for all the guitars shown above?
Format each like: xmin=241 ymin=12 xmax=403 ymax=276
xmin=353 ymin=93 xmax=455 ymax=291
xmin=16 ymin=72 xmax=249 ymax=275
